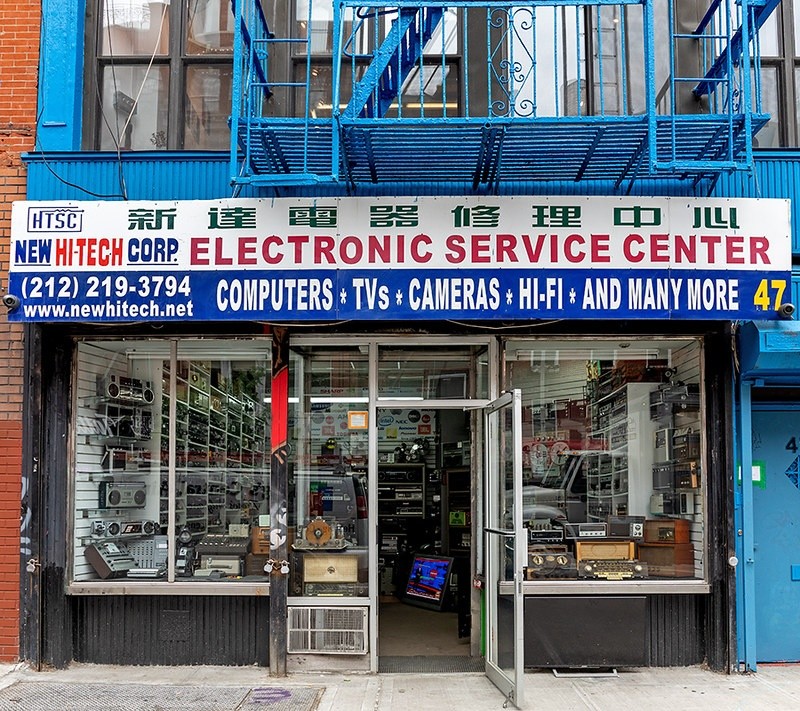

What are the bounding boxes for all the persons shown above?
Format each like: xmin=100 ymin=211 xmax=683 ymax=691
xmin=413 ymin=563 xmax=422 ymax=591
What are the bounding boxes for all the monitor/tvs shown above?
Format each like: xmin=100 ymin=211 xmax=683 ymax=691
xmin=403 ymin=553 xmax=455 ymax=611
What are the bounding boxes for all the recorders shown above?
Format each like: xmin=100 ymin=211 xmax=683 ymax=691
xmin=85 ymin=373 xmax=271 ymax=578
xmin=564 ymin=427 xmax=700 ymax=581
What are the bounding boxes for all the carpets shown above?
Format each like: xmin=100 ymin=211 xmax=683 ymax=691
xmin=377 ymin=655 xmax=485 ymax=674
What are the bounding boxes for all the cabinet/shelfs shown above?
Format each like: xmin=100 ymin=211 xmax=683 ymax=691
xmin=76 ymin=360 xmax=269 ymax=540
xmin=378 ymin=463 xmax=426 ymax=519
xmin=585 ymin=382 xmax=671 ymax=521
xmin=441 ymin=468 xmax=471 ymax=556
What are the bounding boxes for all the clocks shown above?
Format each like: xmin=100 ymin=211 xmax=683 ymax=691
xmin=550 ymin=441 xmax=570 ymax=465
